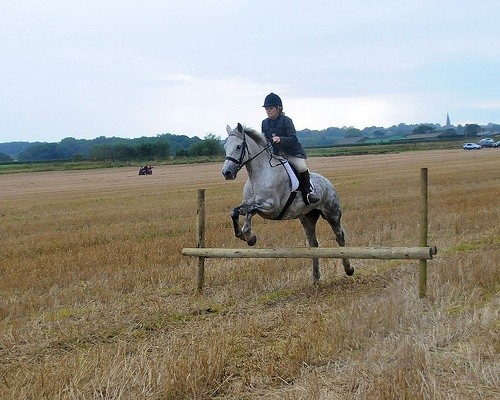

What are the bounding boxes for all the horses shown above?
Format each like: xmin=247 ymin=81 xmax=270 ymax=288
xmin=221 ymin=122 xmax=355 ymax=280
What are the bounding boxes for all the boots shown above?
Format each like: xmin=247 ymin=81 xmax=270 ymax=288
xmin=298 ymin=168 xmax=320 ymax=206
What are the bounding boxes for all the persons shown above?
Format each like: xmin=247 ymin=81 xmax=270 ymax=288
xmin=262 ymin=93 xmax=320 ymax=206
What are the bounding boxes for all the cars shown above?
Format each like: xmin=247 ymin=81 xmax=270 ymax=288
xmin=462 ymin=143 xmax=481 ymax=150
xmin=478 ymin=138 xmax=500 ymax=148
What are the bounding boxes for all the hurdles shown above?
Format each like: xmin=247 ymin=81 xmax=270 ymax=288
xmin=182 ymin=167 xmax=438 ymax=298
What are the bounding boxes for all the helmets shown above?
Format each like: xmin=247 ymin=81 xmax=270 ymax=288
xmin=262 ymin=92 xmax=282 ymax=107
